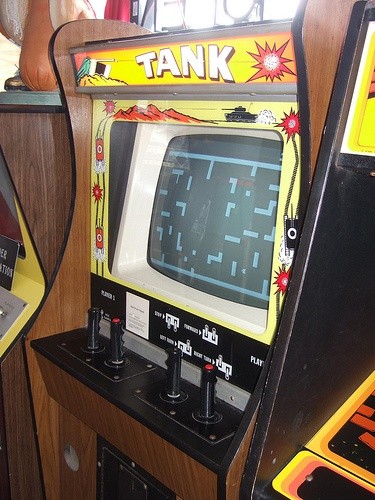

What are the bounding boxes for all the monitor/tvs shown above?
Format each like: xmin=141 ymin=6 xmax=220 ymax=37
xmin=109 ymin=123 xmax=285 ymax=336
